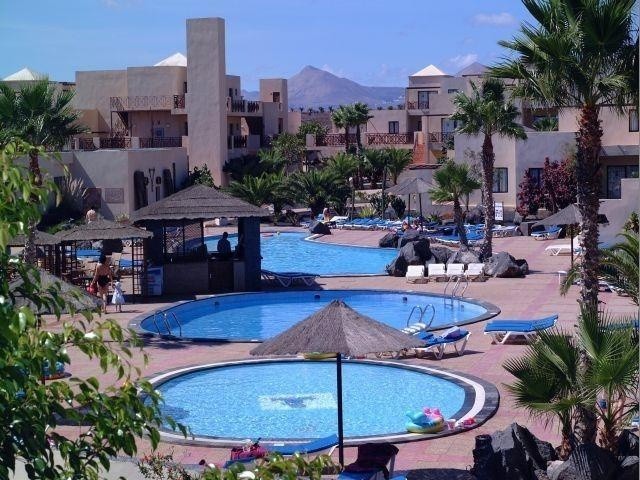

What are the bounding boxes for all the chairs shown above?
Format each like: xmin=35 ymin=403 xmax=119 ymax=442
xmin=337 ymin=442 xmax=409 ymax=480
xmin=557 ymin=267 xmax=615 ymax=292
xmin=225 ymin=434 xmax=339 ymax=469
xmin=544 ymin=242 xmax=609 ymax=256
xmin=483 ymin=314 xmax=558 ymax=346
xmin=531 ymin=227 xmax=562 ymax=241
xmin=261 ymin=268 xmax=326 ymax=290
xmin=415 ymin=326 xmax=471 ymax=359
xmin=406 ymin=264 xmax=490 ymax=283
xmin=300 ymin=213 xmax=520 ymax=236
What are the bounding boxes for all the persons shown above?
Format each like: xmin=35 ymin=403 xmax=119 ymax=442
xmin=90 ymin=254 xmax=113 ymax=314
xmin=323 ymin=207 xmax=331 ymax=221
xmin=217 ymin=232 xmax=231 ymax=255
xmin=112 ymin=281 xmax=125 ymax=312
xmin=86 ymin=206 xmax=96 ymax=224
xmin=402 ymin=220 xmax=422 ymax=233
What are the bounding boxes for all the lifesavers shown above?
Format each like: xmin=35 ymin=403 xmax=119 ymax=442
xmin=406 ymin=407 xmax=445 ymax=432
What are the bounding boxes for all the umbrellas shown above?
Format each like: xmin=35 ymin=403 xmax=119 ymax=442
xmin=9 ymin=267 xmax=107 ymax=385
xmin=531 ymin=204 xmax=610 ymax=268
xmin=249 ymin=301 xmax=427 ymax=472
xmin=396 ymin=178 xmax=438 ymax=232
xmin=383 ymin=178 xmax=413 ymax=226
